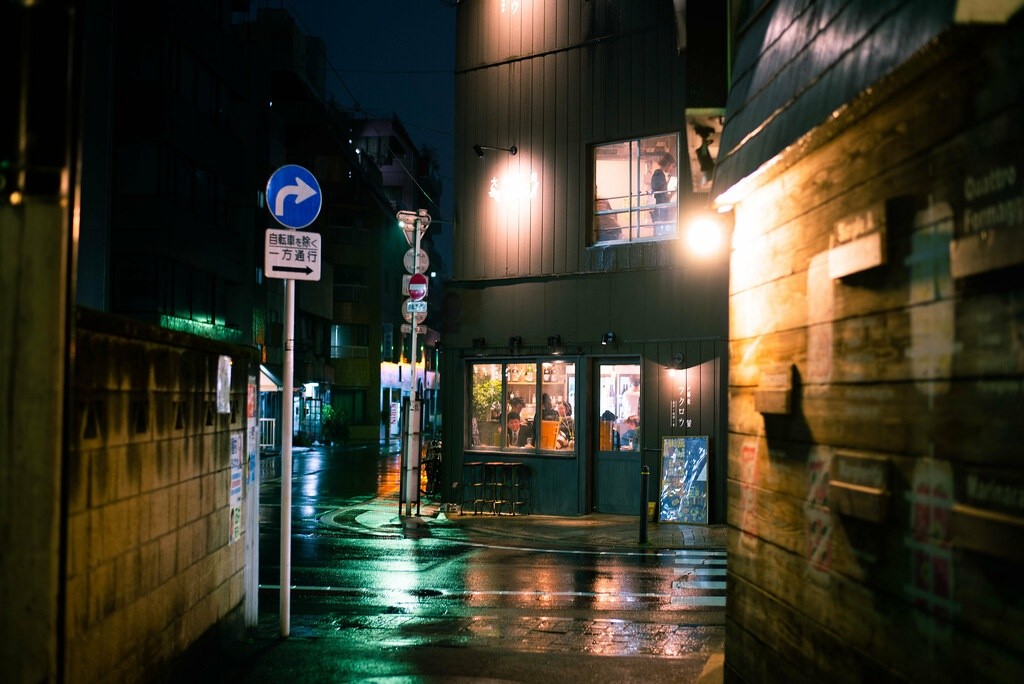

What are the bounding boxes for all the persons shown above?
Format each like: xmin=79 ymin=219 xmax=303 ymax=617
xmin=595 ymin=195 xmax=622 ymax=241
xmin=502 ymin=372 xmax=640 ymax=454
xmin=648 ymin=151 xmax=676 ymax=237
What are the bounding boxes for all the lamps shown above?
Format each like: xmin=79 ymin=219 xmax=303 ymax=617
xmin=473 ymin=144 xmax=517 ymax=159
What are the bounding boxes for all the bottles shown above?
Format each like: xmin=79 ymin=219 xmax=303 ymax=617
xmin=569 ymin=437 xmax=574 ymax=451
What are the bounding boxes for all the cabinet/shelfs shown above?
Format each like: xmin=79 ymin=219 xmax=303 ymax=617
xmin=495 ymin=363 xmax=568 ymax=420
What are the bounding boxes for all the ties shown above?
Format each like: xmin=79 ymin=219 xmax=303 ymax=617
xmin=514 ymin=431 xmax=516 ymax=441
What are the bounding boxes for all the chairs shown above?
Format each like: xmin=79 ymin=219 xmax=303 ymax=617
xmin=600 ymin=421 xmax=613 ymax=451
xmin=541 ymin=419 xmax=562 ymax=450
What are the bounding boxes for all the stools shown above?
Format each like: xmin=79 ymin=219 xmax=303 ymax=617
xmin=481 ymin=461 xmax=511 ymax=515
xmin=499 ymin=462 xmax=530 ymax=516
xmin=461 ymin=462 xmax=491 ymax=516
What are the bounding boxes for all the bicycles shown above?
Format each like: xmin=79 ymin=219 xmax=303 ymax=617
xmin=420 ymin=428 xmax=444 ymax=495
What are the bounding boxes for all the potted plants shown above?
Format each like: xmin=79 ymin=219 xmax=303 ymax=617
xmin=321 ymin=405 xmax=351 ymax=447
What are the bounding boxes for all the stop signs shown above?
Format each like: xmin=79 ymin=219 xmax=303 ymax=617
xmin=408 ymin=272 xmax=427 ymax=301
